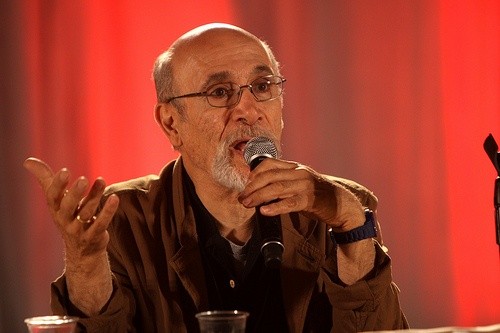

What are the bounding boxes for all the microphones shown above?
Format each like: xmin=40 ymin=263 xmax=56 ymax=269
xmin=242 ymin=136 xmax=285 ymax=267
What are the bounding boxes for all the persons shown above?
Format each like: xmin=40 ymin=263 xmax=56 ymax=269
xmin=24 ymin=22 xmax=410 ymax=333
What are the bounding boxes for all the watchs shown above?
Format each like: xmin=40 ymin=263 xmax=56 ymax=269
xmin=328 ymin=207 xmax=378 ymax=244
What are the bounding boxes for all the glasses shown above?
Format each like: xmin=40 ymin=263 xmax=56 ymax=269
xmin=166 ymin=74 xmax=286 ymax=108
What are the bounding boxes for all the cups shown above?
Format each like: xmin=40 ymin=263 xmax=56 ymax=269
xmin=196 ymin=311 xmax=249 ymax=333
xmin=24 ymin=315 xmax=79 ymax=333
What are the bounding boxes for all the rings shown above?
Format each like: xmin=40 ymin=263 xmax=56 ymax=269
xmin=76 ymin=213 xmax=92 ymax=223
